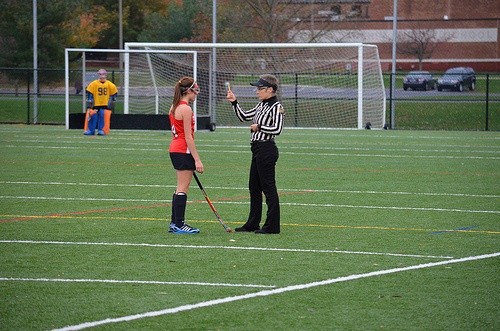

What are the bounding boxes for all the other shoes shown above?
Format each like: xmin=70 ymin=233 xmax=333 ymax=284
xmin=98 ymin=129 xmax=105 ymax=135
xmin=83 ymin=129 xmax=94 ymax=135
xmin=255 ymin=229 xmax=266 ymax=234
xmin=235 ymin=227 xmax=247 ymax=231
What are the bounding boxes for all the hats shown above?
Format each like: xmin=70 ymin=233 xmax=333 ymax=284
xmin=250 ymin=75 xmax=278 ymax=88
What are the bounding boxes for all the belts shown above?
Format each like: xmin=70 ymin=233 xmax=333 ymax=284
xmin=252 ymin=139 xmax=278 ymax=143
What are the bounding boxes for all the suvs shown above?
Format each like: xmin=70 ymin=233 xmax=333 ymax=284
xmin=437 ymin=67 xmax=476 ymax=92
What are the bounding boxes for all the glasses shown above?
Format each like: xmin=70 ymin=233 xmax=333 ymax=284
xmin=191 ymin=87 xmax=201 ymax=94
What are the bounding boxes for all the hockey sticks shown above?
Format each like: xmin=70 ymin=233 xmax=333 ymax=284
xmin=193 ymin=171 xmax=232 ymax=232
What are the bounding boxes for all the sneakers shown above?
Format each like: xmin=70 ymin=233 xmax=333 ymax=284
xmin=169 ymin=223 xmax=200 ymax=234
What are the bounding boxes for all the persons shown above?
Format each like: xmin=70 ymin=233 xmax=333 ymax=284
xmin=226 ymin=74 xmax=285 ymax=234
xmin=168 ymin=77 xmax=204 ymax=234
xmin=83 ymin=69 xmax=118 ymax=136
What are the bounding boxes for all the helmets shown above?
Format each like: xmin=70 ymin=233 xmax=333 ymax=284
xmin=97 ymin=69 xmax=107 ymax=74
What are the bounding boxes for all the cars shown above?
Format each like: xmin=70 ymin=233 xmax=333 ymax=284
xmin=74 ymin=72 xmax=99 ymax=95
xmin=402 ymin=70 xmax=437 ymax=91
